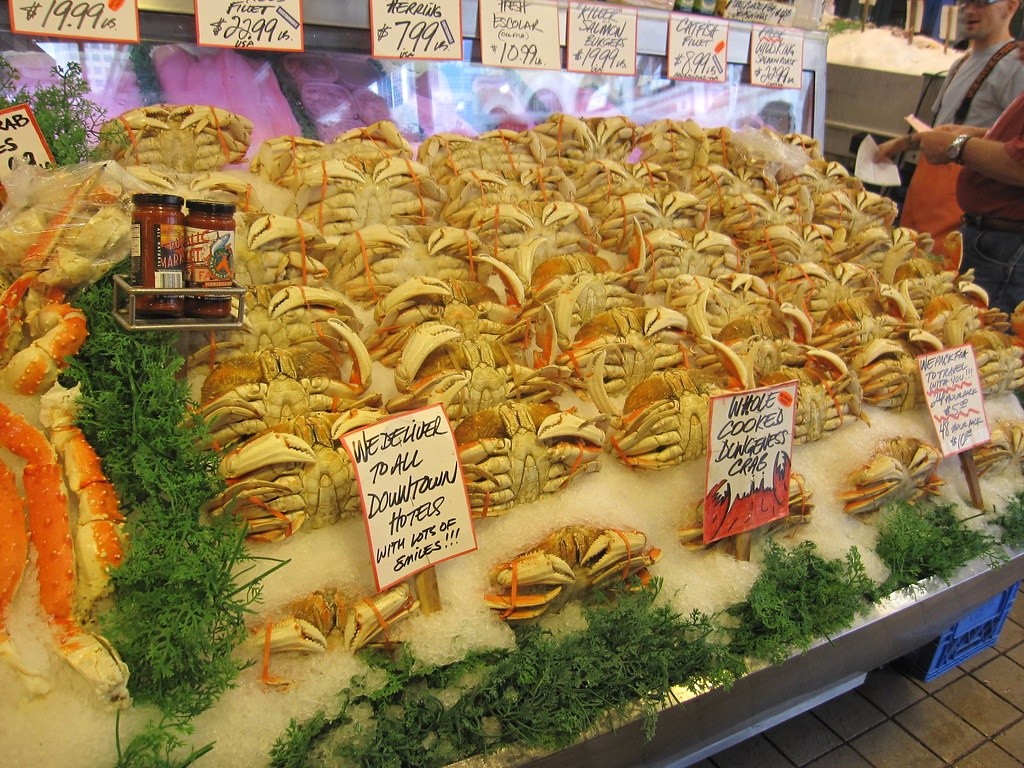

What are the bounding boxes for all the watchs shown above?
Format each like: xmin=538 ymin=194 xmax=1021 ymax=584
xmin=947 ymin=135 xmax=972 ymax=165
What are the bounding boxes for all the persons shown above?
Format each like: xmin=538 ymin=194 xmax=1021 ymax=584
xmin=910 ymin=90 xmax=1024 ymax=337
xmin=873 ymin=0 xmax=1024 ymax=270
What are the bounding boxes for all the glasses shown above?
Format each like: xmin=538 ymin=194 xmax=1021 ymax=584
xmin=957 ymin=0 xmax=1000 ymax=9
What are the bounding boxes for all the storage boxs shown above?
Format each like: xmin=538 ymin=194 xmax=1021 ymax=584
xmin=888 ymin=581 xmax=1021 ymax=683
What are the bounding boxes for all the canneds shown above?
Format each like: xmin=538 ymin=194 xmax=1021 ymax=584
xmin=182 ymin=199 xmax=236 ymax=318
xmin=129 ymin=192 xmax=186 ymax=319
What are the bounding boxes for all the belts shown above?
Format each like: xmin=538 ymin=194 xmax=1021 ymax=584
xmin=967 ymin=214 xmax=1024 ymax=235
